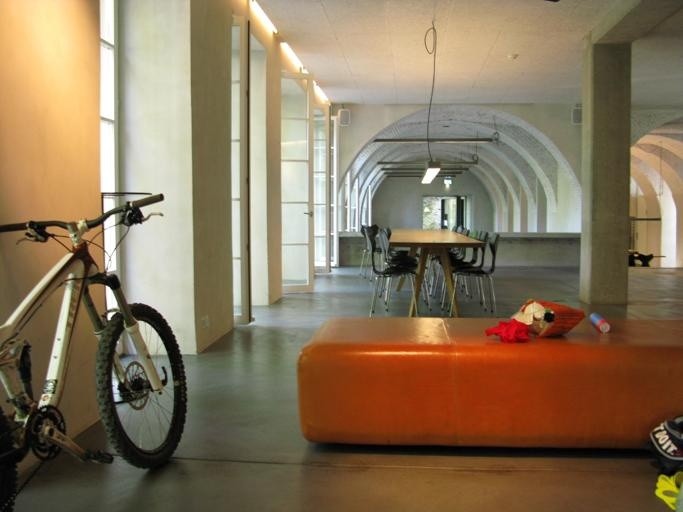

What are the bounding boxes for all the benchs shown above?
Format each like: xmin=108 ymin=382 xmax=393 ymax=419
xmin=296 ymin=316 xmax=683 ymax=455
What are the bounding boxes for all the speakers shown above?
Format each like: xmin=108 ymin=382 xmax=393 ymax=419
xmin=570 ymin=103 xmax=583 ymax=126
xmin=337 ymin=108 xmax=351 ymax=125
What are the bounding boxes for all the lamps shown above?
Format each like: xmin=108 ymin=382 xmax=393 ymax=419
xmin=418 ymin=21 xmax=441 ymax=185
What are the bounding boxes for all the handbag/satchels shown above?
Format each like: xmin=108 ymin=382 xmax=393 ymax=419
xmin=647 ymin=417 xmax=683 ymax=476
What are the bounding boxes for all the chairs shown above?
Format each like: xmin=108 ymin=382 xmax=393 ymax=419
xmin=359 ymin=223 xmax=500 ymax=318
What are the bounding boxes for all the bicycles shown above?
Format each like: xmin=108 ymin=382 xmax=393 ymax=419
xmin=0 ymin=191 xmax=190 ymax=511
